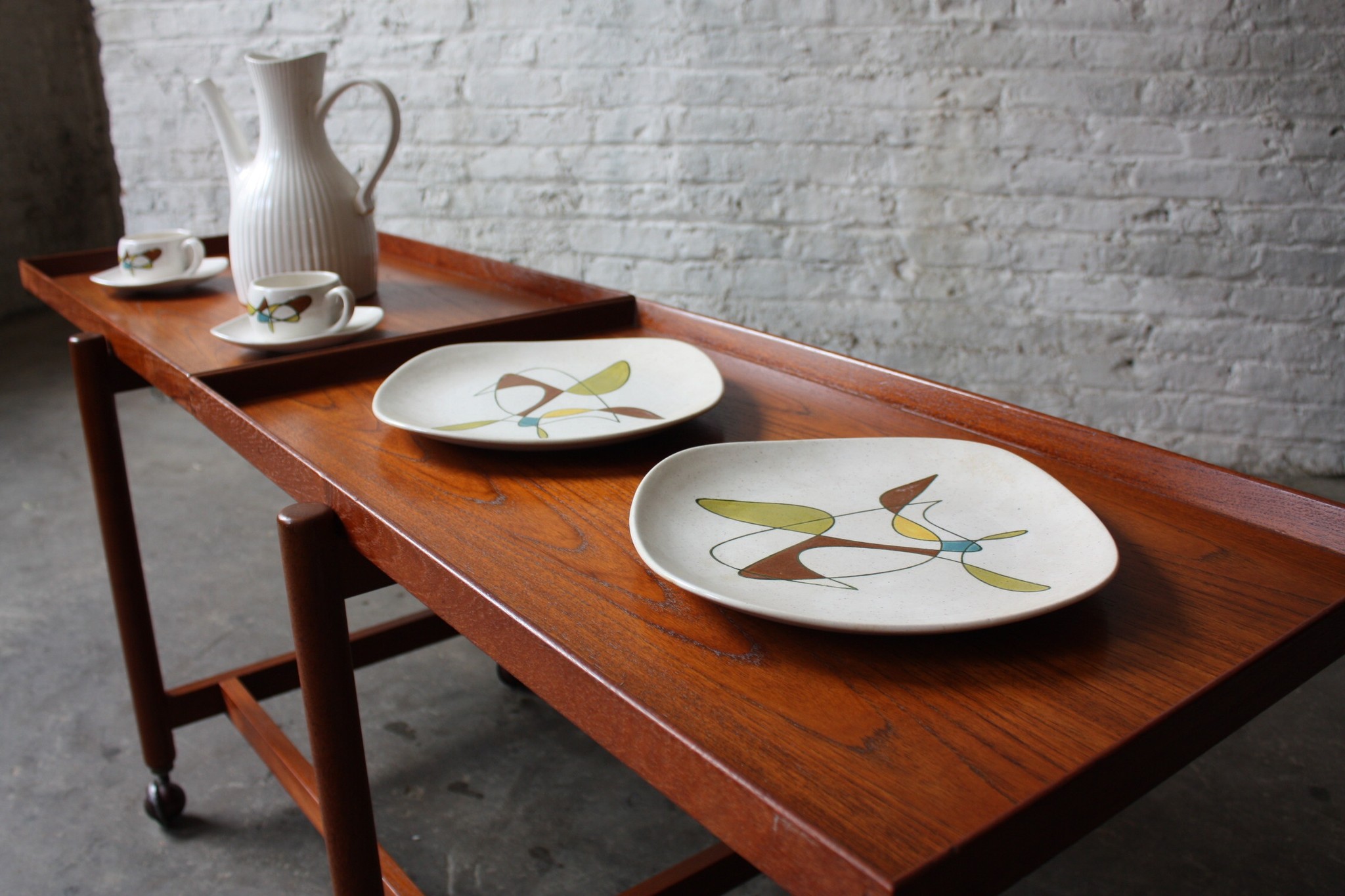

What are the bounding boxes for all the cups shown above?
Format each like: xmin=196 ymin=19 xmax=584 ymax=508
xmin=247 ymin=270 xmax=352 ymax=341
xmin=114 ymin=230 xmax=199 ymax=281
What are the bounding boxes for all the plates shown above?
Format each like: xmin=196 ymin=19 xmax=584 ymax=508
xmin=87 ymin=257 xmax=220 ymax=294
xmin=373 ymin=335 xmax=725 ymax=453
xmin=633 ymin=435 xmax=1120 ymax=635
xmin=210 ymin=305 xmax=385 ymax=355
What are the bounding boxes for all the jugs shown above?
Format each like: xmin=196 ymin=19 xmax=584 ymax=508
xmin=193 ymin=53 xmax=401 ymax=302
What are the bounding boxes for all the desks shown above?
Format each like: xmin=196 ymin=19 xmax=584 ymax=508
xmin=21 ymin=230 xmax=1345 ymax=895
